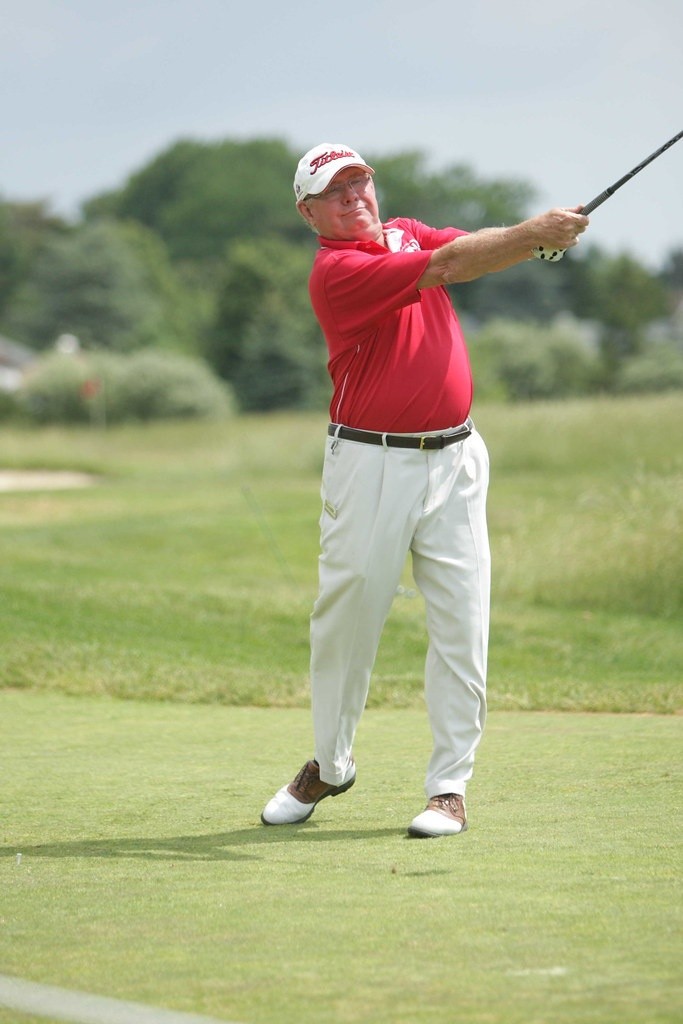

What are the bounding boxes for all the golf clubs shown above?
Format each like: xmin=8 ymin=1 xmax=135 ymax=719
xmin=530 ymin=130 xmax=683 ymax=263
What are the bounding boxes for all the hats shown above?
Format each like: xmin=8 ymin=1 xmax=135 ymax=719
xmin=294 ymin=142 xmax=376 ymax=202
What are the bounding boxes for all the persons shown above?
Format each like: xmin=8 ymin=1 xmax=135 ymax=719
xmin=260 ymin=142 xmax=589 ymax=837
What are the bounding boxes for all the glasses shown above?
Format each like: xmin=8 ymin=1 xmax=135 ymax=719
xmin=304 ymin=173 xmax=371 ymax=202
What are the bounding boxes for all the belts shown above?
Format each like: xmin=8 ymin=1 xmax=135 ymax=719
xmin=327 ymin=422 xmax=474 ymax=452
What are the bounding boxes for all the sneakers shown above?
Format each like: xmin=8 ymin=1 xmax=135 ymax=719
xmin=407 ymin=794 xmax=469 ymax=838
xmin=261 ymin=754 xmax=356 ymax=826
